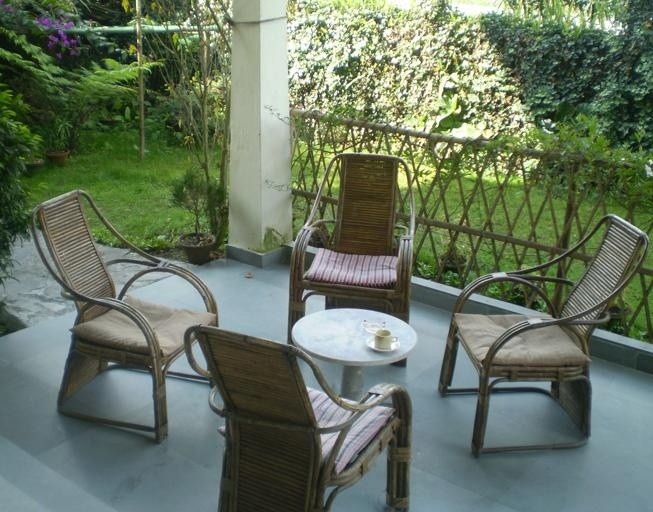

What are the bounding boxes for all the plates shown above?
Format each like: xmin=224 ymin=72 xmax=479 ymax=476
xmin=366 ymin=336 xmax=401 ymax=352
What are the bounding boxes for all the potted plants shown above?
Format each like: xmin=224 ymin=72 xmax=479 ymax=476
xmin=168 ymin=165 xmax=228 ymax=266
xmin=22 ymin=134 xmax=45 ymax=176
xmin=45 ymin=117 xmax=74 ymax=167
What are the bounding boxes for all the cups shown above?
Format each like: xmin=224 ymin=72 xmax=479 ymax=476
xmin=375 ymin=328 xmax=398 ymax=350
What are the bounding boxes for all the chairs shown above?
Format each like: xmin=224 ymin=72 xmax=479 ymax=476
xmin=286 ymin=150 xmax=416 ymax=367
xmin=438 ymin=213 xmax=650 ymax=459
xmin=183 ymin=323 xmax=411 ymax=512
xmin=28 ymin=187 xmax=219 ymax=443
